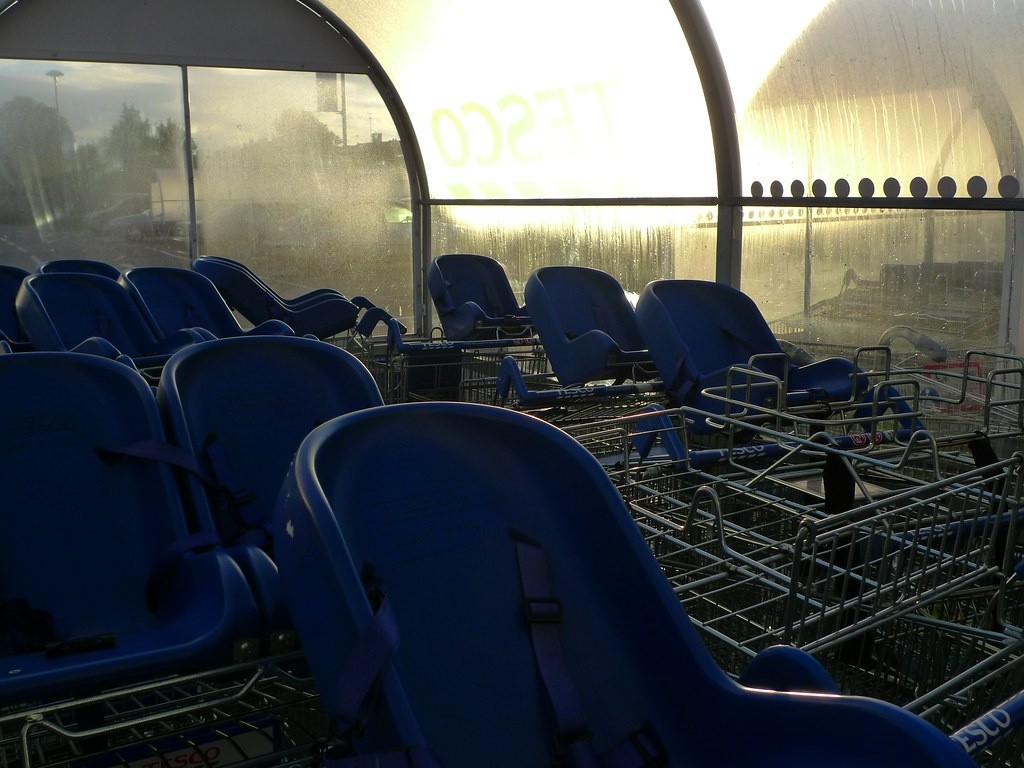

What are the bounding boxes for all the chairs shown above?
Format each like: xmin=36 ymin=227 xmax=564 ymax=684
xmin=0 ymin=254 xmax=974 ymax=768
